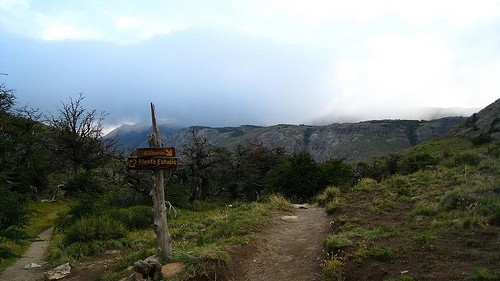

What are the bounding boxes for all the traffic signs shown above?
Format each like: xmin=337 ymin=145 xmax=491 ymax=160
xmin=126 ymin=157 xmax=178 ymax=171
xmin=136 ymin=147 xmax=175 ymax=158
